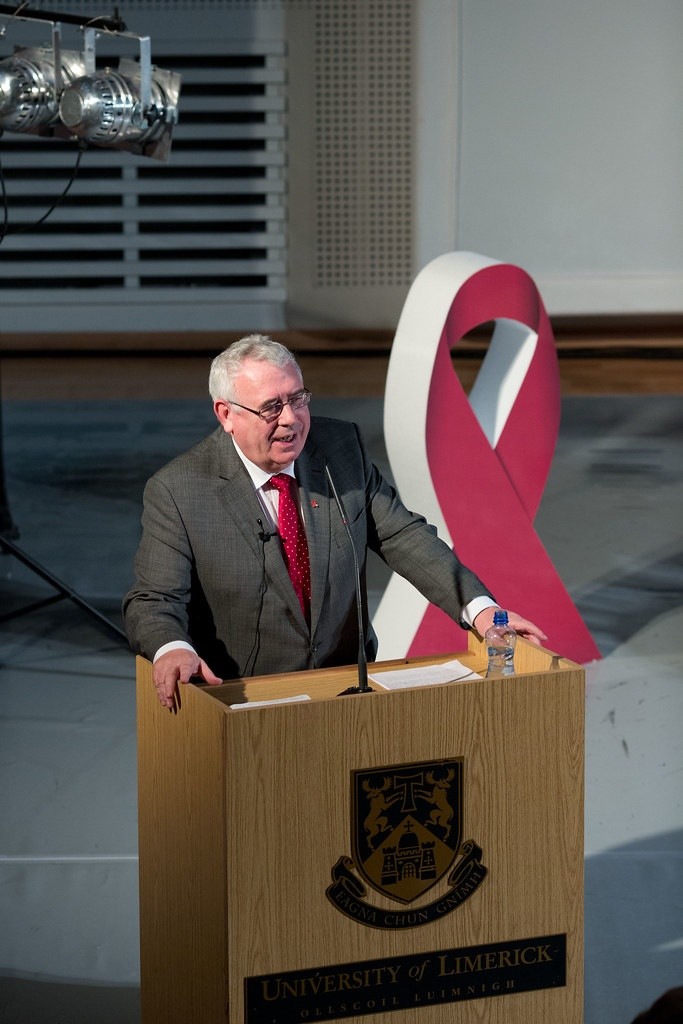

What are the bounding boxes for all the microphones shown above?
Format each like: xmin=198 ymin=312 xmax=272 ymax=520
xmin=323 ymin=465 xmax=375 ymax=698
xmin=256 ymin=519 xmax=267 ymax=541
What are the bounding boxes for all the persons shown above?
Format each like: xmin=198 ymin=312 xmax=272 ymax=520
xmin=122 ymin=334 xmax=548 ymax=708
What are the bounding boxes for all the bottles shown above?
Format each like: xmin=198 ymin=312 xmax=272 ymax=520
xmin=485 ymin=611 xmax=516 ymax=678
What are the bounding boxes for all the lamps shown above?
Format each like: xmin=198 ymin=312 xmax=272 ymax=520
xmin=0 ymin=46 xmax=84 ymax=138
xmin=68 ymin=59 xmax=180 ymax=165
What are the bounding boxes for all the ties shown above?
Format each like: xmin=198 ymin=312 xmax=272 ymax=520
xmin=268 ymin=473 xmax=312 ymax=636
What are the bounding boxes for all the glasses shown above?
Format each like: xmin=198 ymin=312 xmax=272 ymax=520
xmin=227 ymin=386 xmax=312 ymax=420
